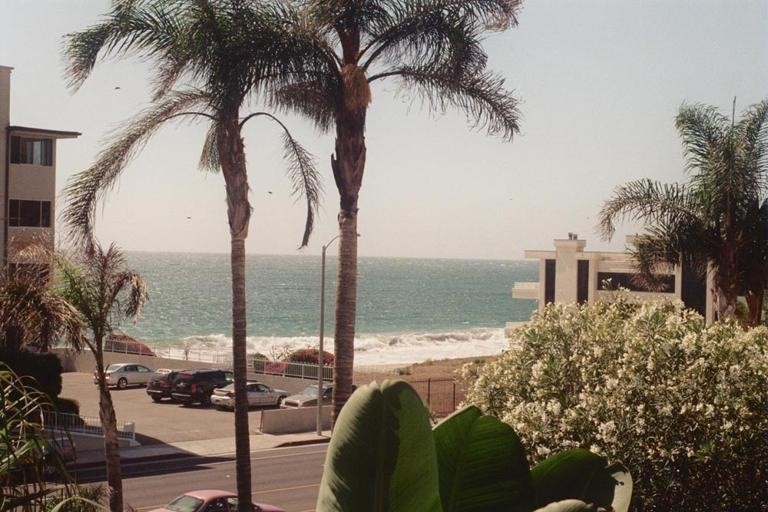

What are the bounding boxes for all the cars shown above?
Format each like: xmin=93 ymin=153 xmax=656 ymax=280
xmin=145 ymin=488 xmax=286 ymax=511
xmin=209 ymin=381 xmax=291 ymax=412
xmin=92 ymin=362 xmax=155 ymax=389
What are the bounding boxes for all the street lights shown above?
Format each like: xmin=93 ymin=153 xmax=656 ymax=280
xmin=314 ymin=232 xmax=364 ymax=434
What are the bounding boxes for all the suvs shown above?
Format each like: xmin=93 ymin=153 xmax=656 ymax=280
xmin=169 ymin=368 xmax=258 ymax=407
xmin=278 ymin=381 xmax=358 ymax=410
xmin=144 ymin=368 xmax=183 ymax=401
